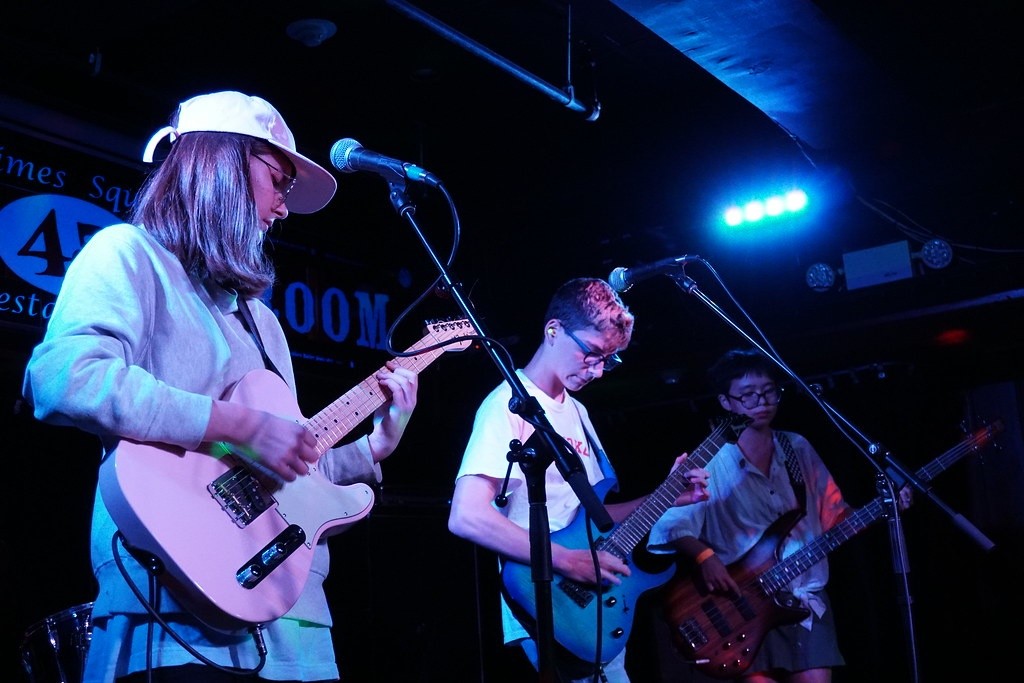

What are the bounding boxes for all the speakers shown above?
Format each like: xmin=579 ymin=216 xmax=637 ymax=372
xmin=326 ymin=503 xmax=483 ymax=683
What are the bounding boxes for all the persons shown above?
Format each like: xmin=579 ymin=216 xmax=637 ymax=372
xmin=20 ymin=90 xmax=420 ymax=683
xmin=447 ymin=278 xmax=710 ymax=682
xmin=646 ymin=349 xmax=912 ymax=683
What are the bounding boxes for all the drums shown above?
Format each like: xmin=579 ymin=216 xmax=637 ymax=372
xmin=17 ymin=600 xmax=94 ymax=681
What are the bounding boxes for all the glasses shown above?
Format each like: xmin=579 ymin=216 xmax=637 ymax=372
xmin=726 ymin=387 xmax=783 ymax=411
xmin=251 ymin=154 xmax=297 ymax=211
xmin=561 ymin=322 xmax=622 ymax=372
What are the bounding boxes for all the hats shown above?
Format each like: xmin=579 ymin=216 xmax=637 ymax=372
xmin=142 ymin=90 xmax=338 ymax=216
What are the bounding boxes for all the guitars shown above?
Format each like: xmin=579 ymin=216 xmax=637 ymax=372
xmin=499 ymin=411 xmax=757 ymax=672
xmin=662 ymin=413 xmax=1007 ymax=679
xmin=98 ymin=315 xmax=478 ymax=638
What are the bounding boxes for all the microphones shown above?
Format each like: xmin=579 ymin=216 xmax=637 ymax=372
xmin=608 ymin=254 xmax=700 ymax=294
xmin=329 ymin=137 xmax=442 ymax=189
